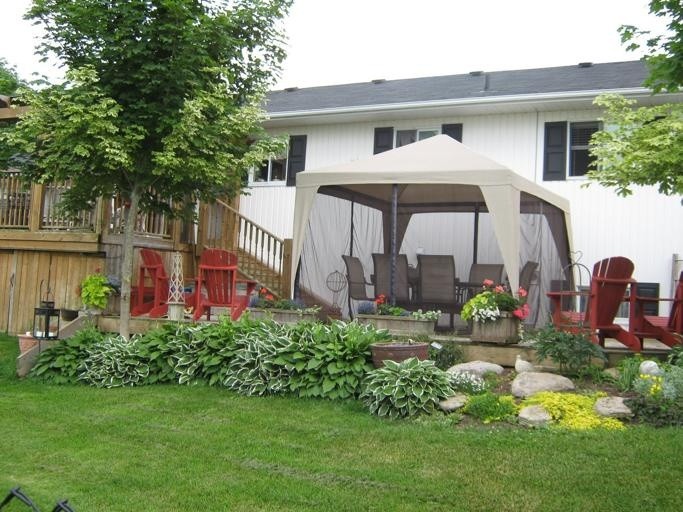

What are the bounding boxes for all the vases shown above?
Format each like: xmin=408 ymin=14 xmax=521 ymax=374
xmin=369 ymin=342 xmax=429 ymax=369
xmin=18 ymin=322 xmax=59 ymax=356
xmin=471 ymin=311 xmax=520 ymax=346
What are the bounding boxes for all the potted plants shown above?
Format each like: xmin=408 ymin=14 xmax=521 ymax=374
xmin=80 ymin=274 xmax=116 ymax=315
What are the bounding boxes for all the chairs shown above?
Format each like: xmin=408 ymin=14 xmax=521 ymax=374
xmin=130 ymin=248 xmax=257 ymax=321
xmin=545 ymin=257 xmax=683 ymax=352
xmin=342 ymin=252 xmax=538 ymax=333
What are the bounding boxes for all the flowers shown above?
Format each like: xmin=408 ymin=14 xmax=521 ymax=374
xmin=249 ymin=284 xmax=274 ymax=308
xmin=356 ymin=292 xmax=442 ymax=321
xmin=461 ymin=277 xmax=529 ymax=340
